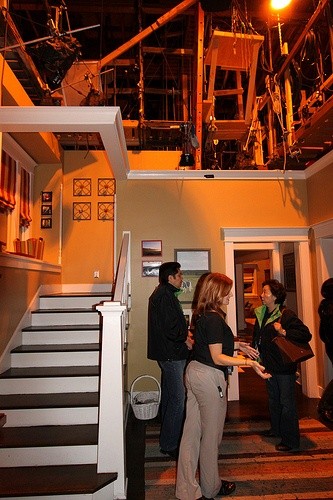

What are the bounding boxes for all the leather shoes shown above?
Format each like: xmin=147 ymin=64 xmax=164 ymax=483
xmin=197 ymin=495 xmax=214 ymax=500
xmin=218 ymin=480 xmax=235 ymax=495
xmin=263 ymin=430 xmax=273 ymax=437
xmin=276 ymin=442 xmax=292 ymax=450
xmin=318 ymin=405 xmax=333 ymax=424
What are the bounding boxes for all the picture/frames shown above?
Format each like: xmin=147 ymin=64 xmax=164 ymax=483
xmin=142 ymin=260 xmax=162 ymax=277
xmin=141 ymin=240 xmax=163 ymax=257
xmin=174 ymin=248 xmax=212 ymax=304
xmin=41 ymin=218 xmax=52 ymax=228
xmin=42 ymin=192 xmax=52 ymax=202
xmin=41 ymin=205 xmax=52 ymax=215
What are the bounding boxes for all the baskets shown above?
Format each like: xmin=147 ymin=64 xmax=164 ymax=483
xmin=131 ymin=375 xmax=161 ymax=419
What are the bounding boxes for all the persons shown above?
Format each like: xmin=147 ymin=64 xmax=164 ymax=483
xmin=315 ymin=278 xmax=333 ymax=424
xmin=147 ymin=262 xmax=194 ymax=455
xmin=175 ymin=271 xmax=272 ymax=500
xmin=252 ymin=279 xmax=312 ymax=450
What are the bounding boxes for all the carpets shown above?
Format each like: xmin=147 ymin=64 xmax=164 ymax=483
xmin=143 ymin=417 xmax=333 ymax=500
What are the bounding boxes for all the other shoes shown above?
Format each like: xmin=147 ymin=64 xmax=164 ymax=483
xmin=161 ymin=448 xmax=178 ymax=458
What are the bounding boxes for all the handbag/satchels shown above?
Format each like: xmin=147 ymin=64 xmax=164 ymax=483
xmin=272 ymin=308 xmax=314 ymax=363
